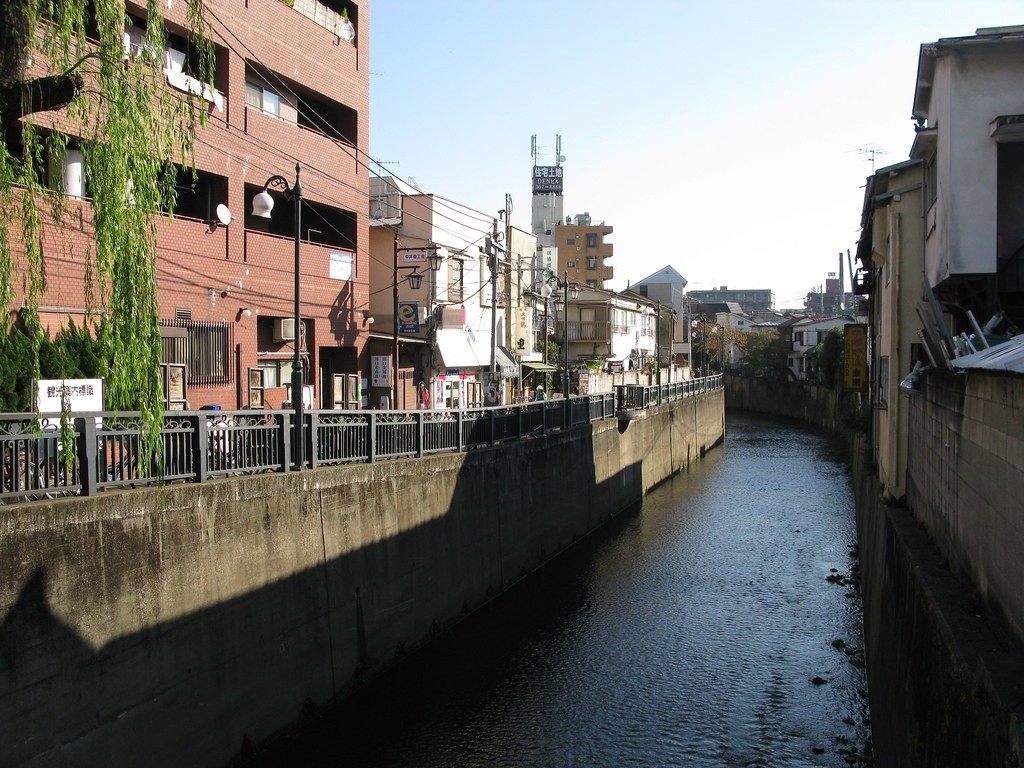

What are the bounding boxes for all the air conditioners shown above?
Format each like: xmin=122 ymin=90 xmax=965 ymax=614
xmin=273 ymin=318 xmax=296 ymax=341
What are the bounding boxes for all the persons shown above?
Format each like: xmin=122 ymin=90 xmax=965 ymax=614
xmin=534 ymin=385 xmax=547 ymax=402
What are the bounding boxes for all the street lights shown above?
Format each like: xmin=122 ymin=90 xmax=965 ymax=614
xmin=710 ymin=324 xmax=724 ymax=388
xmin=392 ymin=228 xmax=441 ymax=407
xmin=249 ymin=162 xmax=305 ymax=471
xmin=642 ymin=297 xmax=662 ymax=407
xmin=691 ymin=315 xmax=704 ymax=394
xmin=541 ymin=268 xmax=571 ymax=401
